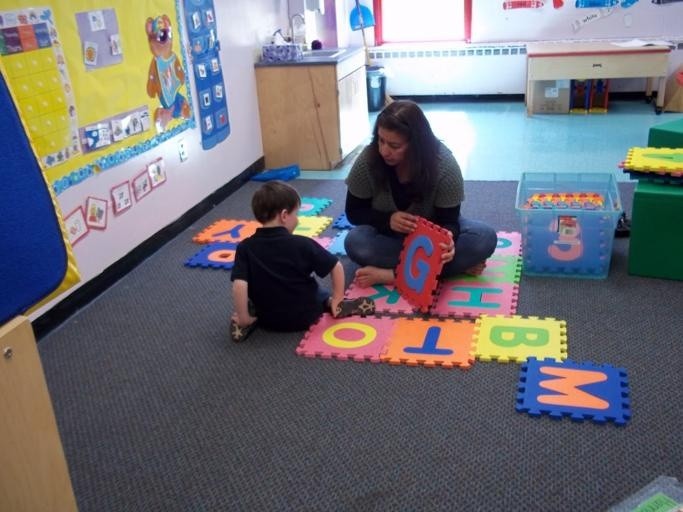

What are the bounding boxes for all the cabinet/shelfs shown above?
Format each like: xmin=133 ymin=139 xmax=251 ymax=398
xmin=255 ymin=50 xmax=370 ymax=171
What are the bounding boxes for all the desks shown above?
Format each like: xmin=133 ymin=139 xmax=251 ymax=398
xmin=524 ymin=40 xmax=672 ymax=116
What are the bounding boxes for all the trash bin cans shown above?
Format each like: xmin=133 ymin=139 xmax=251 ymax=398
xmin=367 ymin=65 xmax=386 ymax=112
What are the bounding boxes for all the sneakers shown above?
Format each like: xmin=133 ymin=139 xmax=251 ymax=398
xmin=229 ymin=319 xmax=250 ymax=343
xmin=335 ymin=296 xmax=375 ymax=318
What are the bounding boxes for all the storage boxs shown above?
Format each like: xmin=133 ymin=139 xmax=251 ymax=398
xmin=515 ymin=172 xmax=624 ymax=281
xmin=533 ymin=80 xmax=571 ymax=114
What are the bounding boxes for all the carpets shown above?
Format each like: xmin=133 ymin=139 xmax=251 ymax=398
xmin=36 ymin=178 xmax=683 ymax=512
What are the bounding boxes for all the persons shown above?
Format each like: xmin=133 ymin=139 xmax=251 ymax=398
xmin=228 ymin=179 xmax=375 ymax=344
xmin=342 ymin=99 xmax=498 ymax=289
